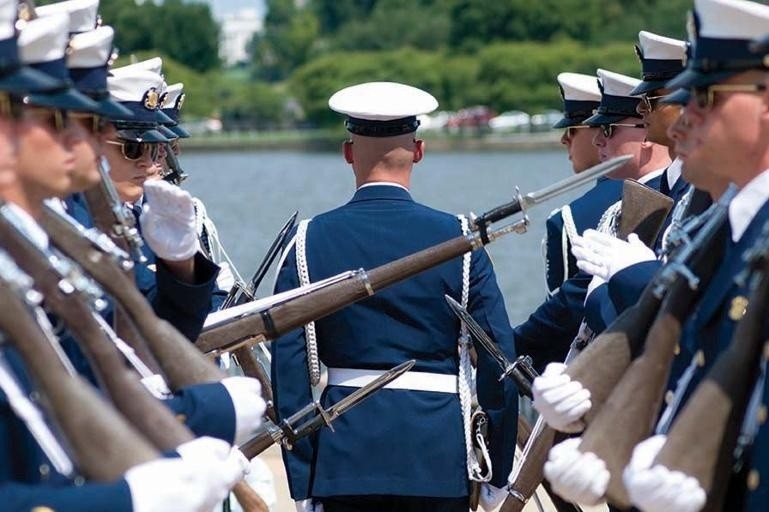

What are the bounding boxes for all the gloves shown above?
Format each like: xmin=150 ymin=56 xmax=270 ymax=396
xmin=293 ymin=497 xmax=325 ymax=512
xmin=571 ymin=227 xmax=659 ymax=284
xmin=539 ymin=436 xmax=613 ymax=508
xmin=138 ymin=372 xmax=175 ymax=400
xmin=123 ymin=433 xmax=253 ymax=512
xmin=138 ymin=176 xmax=201 ymax=264
xmin=583 ymin=274 xmax=609 ymax=308
xmin=218 ymin=371 xmax=269 ymax=447
xmin=216 ymin=260 xmax=237 ymax=293
xmin=620 ymin=433 xmax=709 ymax=512
xmin=475 ymin=479 xmax=514 ymax=512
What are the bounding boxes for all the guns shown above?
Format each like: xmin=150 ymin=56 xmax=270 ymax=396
xmin=1 ymin=275 xmax=161 ymax=486
xmin=160 ymin=144 xmax=277 ymax=425
xmin=499 ymin=176 xmax=674 ymax=511
xmin=194 ymin=152 xmax=634 ymax=364
xmin=577 ymin=207 xmax=728 ymax=511
xmin=238 ymin=358 xmax=417 ymax=461
xmin=444 ymin=293 xmax=540 ymax=403
xmin=36 ymin=202 xmax=226 ymax=394
xmin=649 ymin=267 xmax=764 ymax=511
xmin=2 ymin=213 xmax=196 ymax=452
xmin=85 ymin=155 xmax=163 ymax=376
xmin=556 ymin=201 xmax=715 ymax=428
xmin=204 ymin=211 xmax=298 ymax=369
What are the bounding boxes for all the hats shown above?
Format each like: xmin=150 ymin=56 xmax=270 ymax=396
xmin=628 ymin=30 xmax=693 ymax=97
xmin=658 ymin=0 xmax=769 ymax=104
xmin=326 ymin=79 xmax=442 ymax=138
xmin=107 ymin=56 xmax=193 ymax=143
xmin=581 ymin=66 xmax=645 ymax=126
xmin=552 ymin=71 xmax=604 ymax=130
xmin=0 ymin=1 xmax=112 ymax=113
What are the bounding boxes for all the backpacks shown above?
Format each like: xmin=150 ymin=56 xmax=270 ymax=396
xmin=529 ymin=360 xmax=594 ymax=436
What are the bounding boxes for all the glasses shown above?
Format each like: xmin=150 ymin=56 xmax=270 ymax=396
xmin=105 ymin=139 xmax=160 ymax=164
xmin=566 ymin=125 xmax=600 ymax=139
xmin=0 ymin=90 xmax=19 ymax=120
xmin=640 ymin=94 xmax=669 ymax=113
xmin=692 ymin=81 xmax=767 ymax=114
xmin=600 ymin=122 xmax=646 ymax=139
xmin=20 ymin=104 xmax=66 ymax=134
xmin=66 ymin=110 xmax=104 ymax=136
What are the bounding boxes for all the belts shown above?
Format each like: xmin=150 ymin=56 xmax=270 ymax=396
xmin=325 ymin=365 xmax=460 ymax=395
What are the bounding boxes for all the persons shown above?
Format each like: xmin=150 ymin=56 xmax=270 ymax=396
xmin=533 ymin=1 xmax=769 ymax=512
xmin=0 ymin=0 xmax=267 ymax=512
xmin=267 ymin=75 xmax=520 ymax=512
xmin=468 ymin=69 xmax=672 ymax=373
